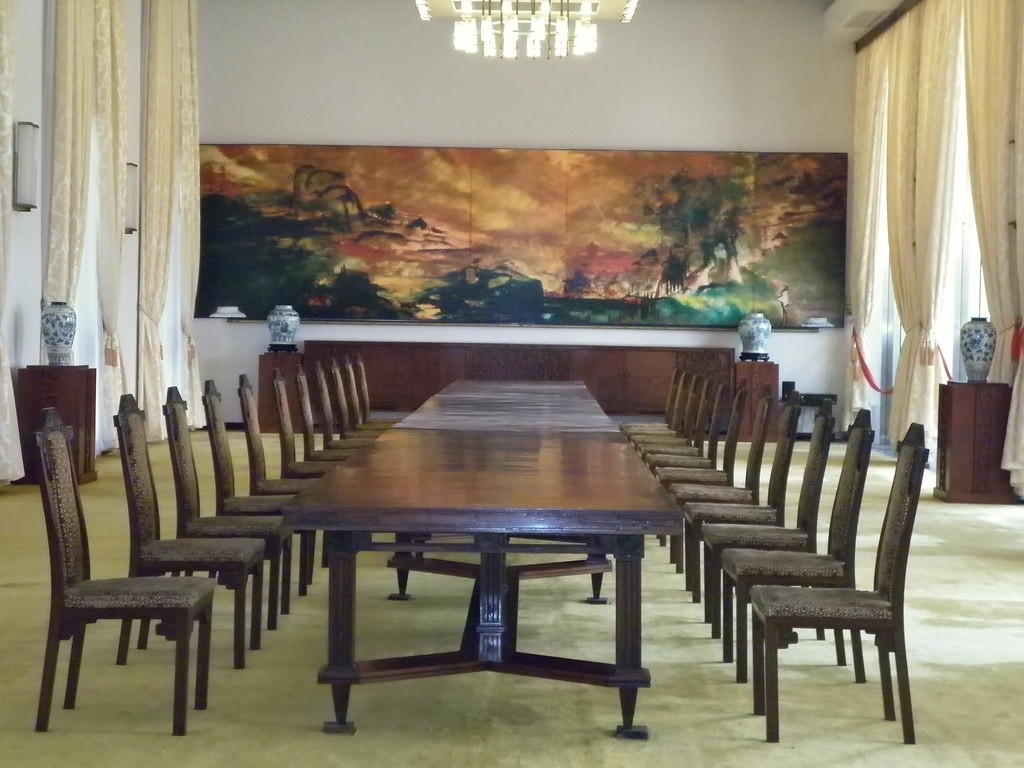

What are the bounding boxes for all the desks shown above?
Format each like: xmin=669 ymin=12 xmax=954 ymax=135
xmin=283 ymin=381 xmax=685 ymax=739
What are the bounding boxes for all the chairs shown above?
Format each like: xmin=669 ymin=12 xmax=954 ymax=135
xmin=115 ymin=394 xmax=268 ymax=670
xmin=31 ymin=405 xmax=216 ymax=736
xmin=201 ymin=356 xmax=401 ymax=594
xmin=162 ymin=386 xmax=292 ymax=631
xmin=718 ymin=408 xmax=875 ymax=682
xmin=619 ymin=369 xmax=837 ymax=641
xmin=753 ymin=422 xmax=931 ymax=744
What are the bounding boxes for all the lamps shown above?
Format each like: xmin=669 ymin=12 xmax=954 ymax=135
xmin=125 ymin=162 xmax=139 ymax=235
xmin=13 ymin=121 xmax=39 ymax=212
xmin=414 ymin=0 xmax=638 ymax=60
